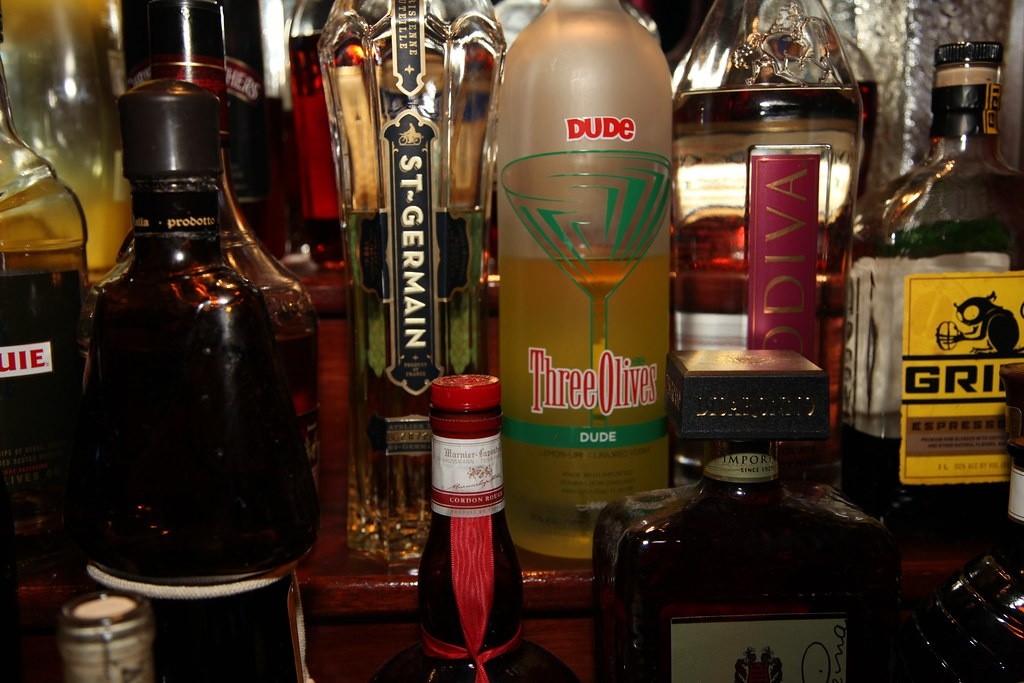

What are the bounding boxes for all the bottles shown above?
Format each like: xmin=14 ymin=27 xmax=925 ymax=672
xmin=53 ymin=590 xmax=155 ymax=683
xmin=671 ymin=0 xmax=877 ymax=465
xmin=838 ymin=41 xmax=1024 ymax=540
xmin=497 ymin=0 xmax=671 ymax=560
xmin=365 ymin=373 xmax=581 ymax=683
xmin=288 ymin=0 xmax=505 ymax=576
xmin=913 ymin=363 xmax=1024 ymax=683
xmin=0 ymin=0 xmax=1024 ymax=274
xmin=593 ymin=348 xmax=897 ymax=683
xmin=85 ymin=80 xmax=319 ymax=600
xmin=0 ymin=0 xmax=89 ymax=580
